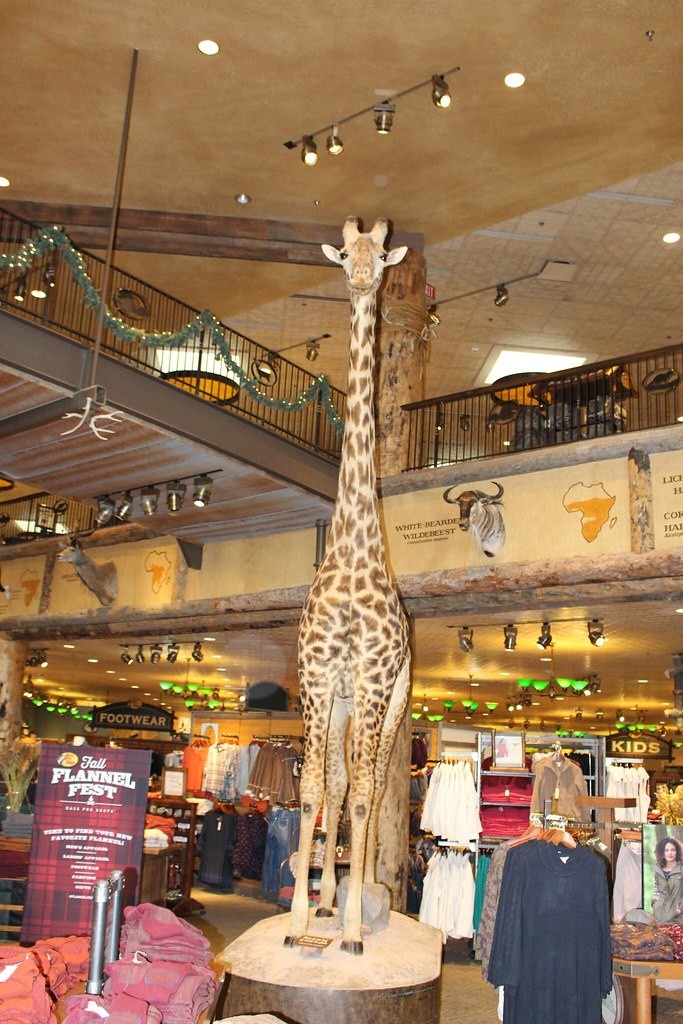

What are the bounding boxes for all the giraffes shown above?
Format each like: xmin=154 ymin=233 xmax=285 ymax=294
xmin=284 ymin=217 xmax=415 ymax=959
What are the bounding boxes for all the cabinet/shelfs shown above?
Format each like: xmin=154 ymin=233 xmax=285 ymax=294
xmin=146 ymin=796 xmax=208 ymax=916
xmin=478 ymin=769 xmax=595 ymax=850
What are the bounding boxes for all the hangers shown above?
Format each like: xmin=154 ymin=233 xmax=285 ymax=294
xmin=511 ymin=810 xmax=599 ymax=848
xmin=192 ymin=734 xmax=291 ymax=749
xmin=212 ymin=800 xmax=233 ymax=813
xmin=246 ymin=801 xmax=300 ymax=815
xmin=550 ymin=742 xmax=564 ymax=764
xmin=410 ymin=756 xmax=494 ymax=858
xmin=608 ymin=758 xmax=644 ymax=769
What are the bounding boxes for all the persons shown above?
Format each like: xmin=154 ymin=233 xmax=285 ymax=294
xmin=651 ymin=837 xmax=683 ymax=925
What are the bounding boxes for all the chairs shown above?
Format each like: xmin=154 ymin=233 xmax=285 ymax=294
xmin=276 ymin=824 xmax=340 ymax=912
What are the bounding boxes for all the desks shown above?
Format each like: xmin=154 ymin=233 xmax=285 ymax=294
xmin=160 ymin=371 xmax=241 ymax=409
xmin=612 ymin=956 xmax=683 ymax=1024
xmin=489 ymin=371 xmax=552 ymax=410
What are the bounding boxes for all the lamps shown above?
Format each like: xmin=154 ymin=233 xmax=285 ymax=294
xmin=43 ymin=268 xmax=56 ymax=287
xmin=493 ymin=285 xmax=509 ymax=307
xmin=305 ymin=339 xmax=321 ymax=361
xmin=437 ymin=413 xmax=497 ymax=435
xmin=411 ymin=621 xmax=683 ymax=750
xmin=267 ymin=355 xmax=280 ymax=373
xmin=428 ymin=305 xmax=441 ymax=327
xmin=119 ymin=642 xmax=220 ymax=712
xmin=300 ymin=76 xmax=451 ymax=167
xmin=94 ymin=474 xmax=214 ymax=524
xmin=25 ymin=648 xmax=94 ymax=721
xmin=15 ymin=268 xmax=27 ymax=301
